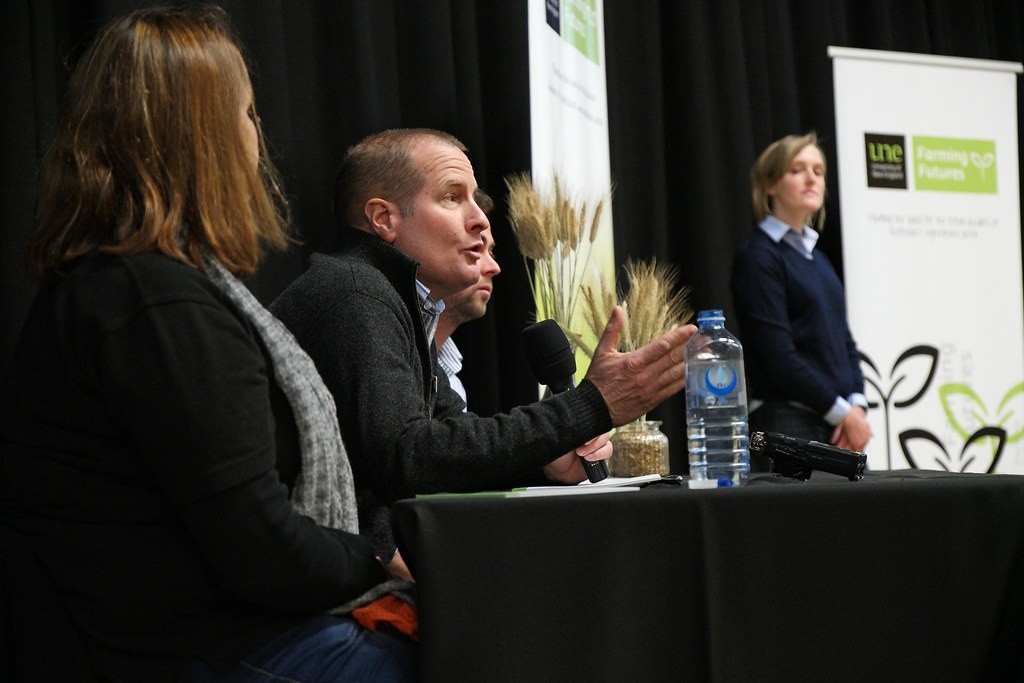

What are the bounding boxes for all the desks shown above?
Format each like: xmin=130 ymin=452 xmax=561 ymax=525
xmin=391 ymin=464 xmax=1023 ymax=683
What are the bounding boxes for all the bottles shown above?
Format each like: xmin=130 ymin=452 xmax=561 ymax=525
xmin=683 ymin=308 xmax=750 ymax=489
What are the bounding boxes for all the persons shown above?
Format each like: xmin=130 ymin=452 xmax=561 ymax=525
xmin=730 ymin=135 xmax=871 ymax=480
xmin=0 ymin=8 xmax=417 ymax=683
xmin=267 ymin=128 xmax=714 ymax=582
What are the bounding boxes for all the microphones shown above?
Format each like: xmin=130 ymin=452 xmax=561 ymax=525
xmin=521 ymin=320 xmax=609 ymax=483
